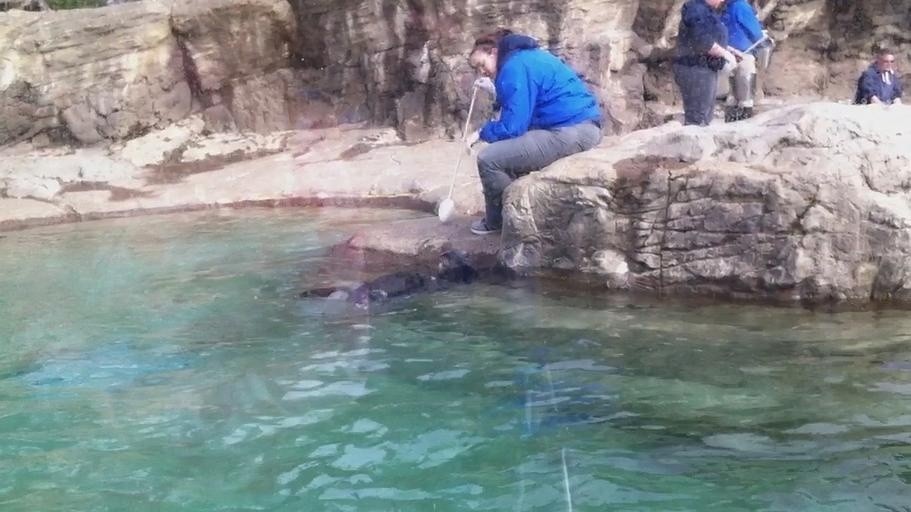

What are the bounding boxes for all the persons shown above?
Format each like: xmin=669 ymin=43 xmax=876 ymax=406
xmin=856 ymin=49 xmax=903 ymax=105
xmin=675 ymin=0 xmax=774 ymax=125
xmin=466 ymin=27 xmax=602 ymax=234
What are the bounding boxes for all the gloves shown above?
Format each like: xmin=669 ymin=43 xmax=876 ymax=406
xmin=473 ymin=76 xmax=496 ymax=95
xmin=465 ymin=128 xmax=481 ymax=156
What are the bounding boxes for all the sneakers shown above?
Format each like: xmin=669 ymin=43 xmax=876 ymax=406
xmin=470 ymin=215 xmax=502 ymax=235
